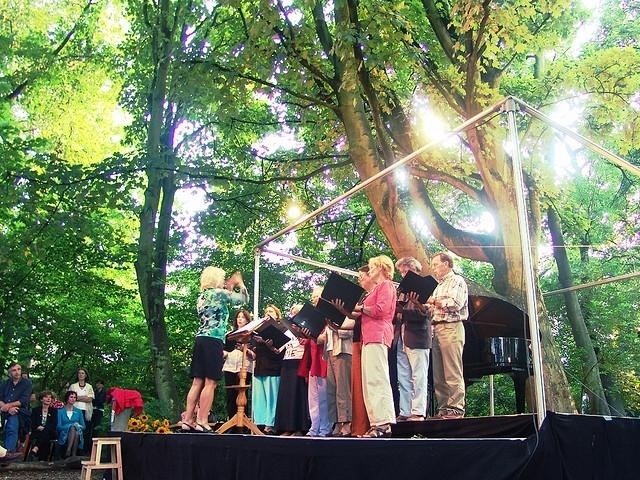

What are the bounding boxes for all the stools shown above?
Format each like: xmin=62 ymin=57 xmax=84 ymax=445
xmin=79 ymin=436 xmax=125 ymax=480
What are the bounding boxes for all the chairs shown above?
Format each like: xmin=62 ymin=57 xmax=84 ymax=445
xmin=26 ymin=393 xmax=36 ymax=410
xmin=22 ymin=430 xmax=58 ymax=462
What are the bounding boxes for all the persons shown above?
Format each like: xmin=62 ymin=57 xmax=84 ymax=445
xmin=395 ymin=256 xmax=432 ymax=420
xmin=407 ymin=254 xmax=471 ymax=417
xmin=224 ymin=310 xmax=255 ymax=431
xmin=0 ymin=361 xmax=107 ymax=468
xmin=250 ymin=285 xmax=355 ymax=437
xmin=180 ymin=263 xmax=251 ymax=434
xmin=330 ymin=255 xmax=396 ymax=437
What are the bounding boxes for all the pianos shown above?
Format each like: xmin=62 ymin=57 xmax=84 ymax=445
xmin=429 ymin=294 xmax=542 ymax=414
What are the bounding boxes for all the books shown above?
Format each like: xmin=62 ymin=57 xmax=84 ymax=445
xmin=397 ymin=269 xmax=438 ymax=305
xmin=224 ymin=330 xmax=249 ymax=352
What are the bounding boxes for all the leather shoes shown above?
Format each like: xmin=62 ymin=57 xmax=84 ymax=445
xmin=396 ymin=412 xmax=465 ymax=422
xmin=0 ymin=452 xmax=21 ymax=463
xmin=264 ymin=426 xmax=350 ymax=437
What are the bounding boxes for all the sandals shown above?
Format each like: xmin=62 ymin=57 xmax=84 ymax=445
xmin=179 ymin=423 xmax=213 ymax=433
xmin=357 ymin=425 xmax=392 ymax=437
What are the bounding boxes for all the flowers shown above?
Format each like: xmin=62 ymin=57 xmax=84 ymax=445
xmin=127 ymin=414 xmax=172 ymax=435
